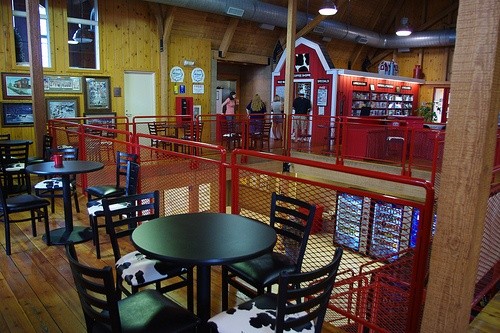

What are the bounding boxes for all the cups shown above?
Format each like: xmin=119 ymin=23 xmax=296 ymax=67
xmin=50 ymin=155 xmax=63 ymax=168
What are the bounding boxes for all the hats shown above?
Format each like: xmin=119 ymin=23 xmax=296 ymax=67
xmin=298 ymin=89 xmax=304 ymax=94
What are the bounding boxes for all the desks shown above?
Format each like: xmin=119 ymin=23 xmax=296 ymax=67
xmin=26 ymin=161 xmax=105 ymax=244
xmin=131 ymin=212 xmax=277 ymax=320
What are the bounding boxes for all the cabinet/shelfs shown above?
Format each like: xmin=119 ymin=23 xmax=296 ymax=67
xmin=351 ymin=90 xmax=413 ymax=117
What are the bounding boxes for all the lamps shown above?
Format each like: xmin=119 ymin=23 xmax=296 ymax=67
xmin=396 ymin=16 xmax=412 ymax=36
xmin=319 ymin=0 xmax=337 ymax=15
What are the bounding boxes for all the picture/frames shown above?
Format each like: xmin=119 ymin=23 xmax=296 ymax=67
xmin=45 ymin=96 xmax=79 ymax=124
xmin=84 ymin=112 xmax=117 ymax=137
xmin=1 ymin=72 xmax=32 ymax=100
xmin=43 ymin=75 xmax=82 ymax=93
xmin=0 ymin=104 xmax=34 ymax=127
xmin=84 ymin=76 xmax=112 ymax=115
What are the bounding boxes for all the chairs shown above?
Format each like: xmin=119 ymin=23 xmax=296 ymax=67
xmin=0 ymin=123 xmax=343 ymax=333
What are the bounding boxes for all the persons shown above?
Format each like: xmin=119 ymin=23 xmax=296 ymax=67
xmin=220 ymin=90 xmax=238 ymax=133
xmin=271 ymin=95 xmax=283 ymax=140
xmin=292 ymin=89 xmax=312 ymax=142
xmin=246 ymin=94 xmax=267 ymax=149
